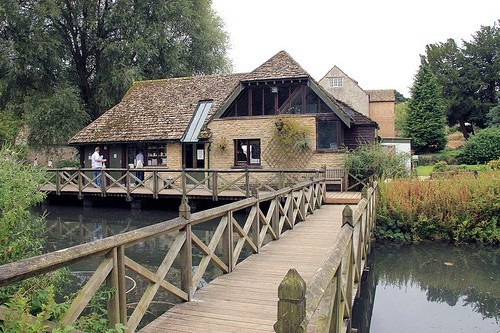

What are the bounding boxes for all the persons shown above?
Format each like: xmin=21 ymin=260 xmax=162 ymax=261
xmin=34 ymin=158 xmax=38 ymax=167
xmin=91 ymin=146 xmax=108 ymax=188
xmin=48 ymin=159 xmax=53 ymax=167
xmin=134 ymin=149 xmax=147 ymax=187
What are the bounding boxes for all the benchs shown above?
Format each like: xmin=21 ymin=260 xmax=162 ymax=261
xmin=305 ymin=168 xmax=345 ymax=192
